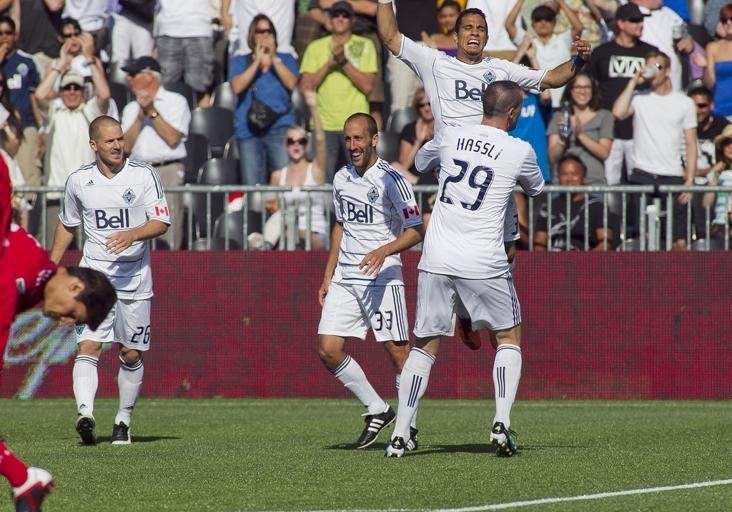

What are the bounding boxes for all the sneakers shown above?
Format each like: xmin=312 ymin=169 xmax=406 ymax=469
xmin=355 ymin=402 xmax=417 ymax=457
xmin=111 ymin=421 xmax=131 ymax=444
xmin=75 ymin=415 xmax=96 ymax=444
xmin=458 ymin=318 xmax=480 ymax=350
xmin=489 ymin=422 xmax=517 ymax=456
xmin=12 ymin=467 xmax=54 ymax=512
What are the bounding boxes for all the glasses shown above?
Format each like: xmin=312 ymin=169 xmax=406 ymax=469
xmin=419 ymin=102 xmax=430 ymax=107
xmin=287 ymin=138 xmax=307 ymax=145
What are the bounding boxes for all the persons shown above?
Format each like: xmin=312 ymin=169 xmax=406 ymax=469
xmin=1 ymin=150 xmax=56 ymax=512
xmin=381 ymin=78 xmax=547 ymax=457
xmin=315 ymin=112 xmax=427 ymax=451
xmin=0 ymin=0 xmax=732 ymax=252
xmin=48 ymin=113 xmax=172 ymax=448
xmin=376 ymin=0 xmax=595 ymax=352
xmin=0 ymin=216 xmax=117 ymax=365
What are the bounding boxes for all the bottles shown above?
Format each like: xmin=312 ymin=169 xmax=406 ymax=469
xmin=560 ymin=101 xmax=572 ymax=136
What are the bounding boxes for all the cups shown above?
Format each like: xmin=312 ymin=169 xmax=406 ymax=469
xmin=642 ymin=66 xmax=658 ymax=79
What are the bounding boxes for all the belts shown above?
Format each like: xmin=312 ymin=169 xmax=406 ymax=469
xmin=153 ymin=160 xmax=180 ymax=166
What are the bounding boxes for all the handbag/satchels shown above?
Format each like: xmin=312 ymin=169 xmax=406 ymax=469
xmin=247 ymin=99 xmax=279 ymax=136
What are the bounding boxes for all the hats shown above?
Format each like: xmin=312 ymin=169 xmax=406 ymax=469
xmin=616 ymin=3 xmax=651 ymax=19
xmin=331 ymin=2 xmax=352 ymax=15
xmin=122 ymin=57 xmax=161 ymax=73
xmin=714 ymin=124 xmax=732 ymax=147
xmin=532 ymin=6 xmax=553 ymax=17
xmin=60 ymin=72 xmax=84 ymax=88
